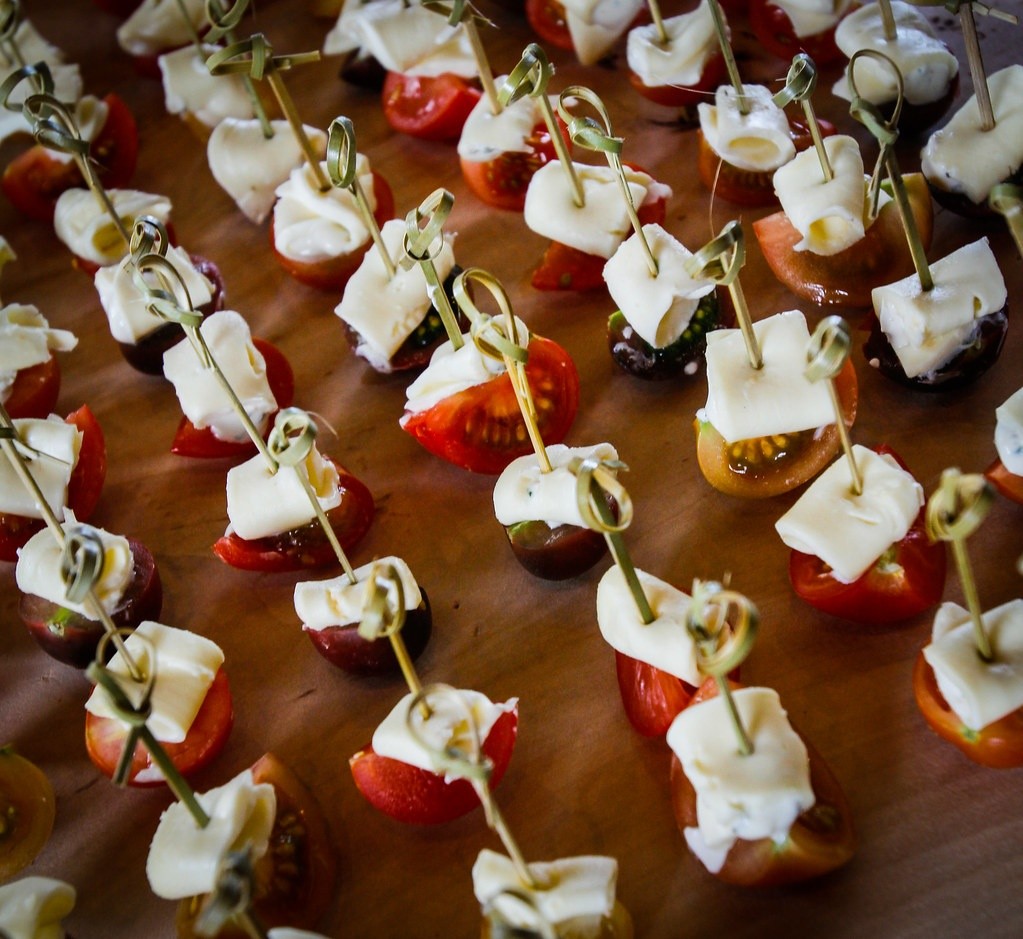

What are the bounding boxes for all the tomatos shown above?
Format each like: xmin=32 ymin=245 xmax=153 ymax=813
xmin=0 ymin=0 xmax=1023 ymax=939
